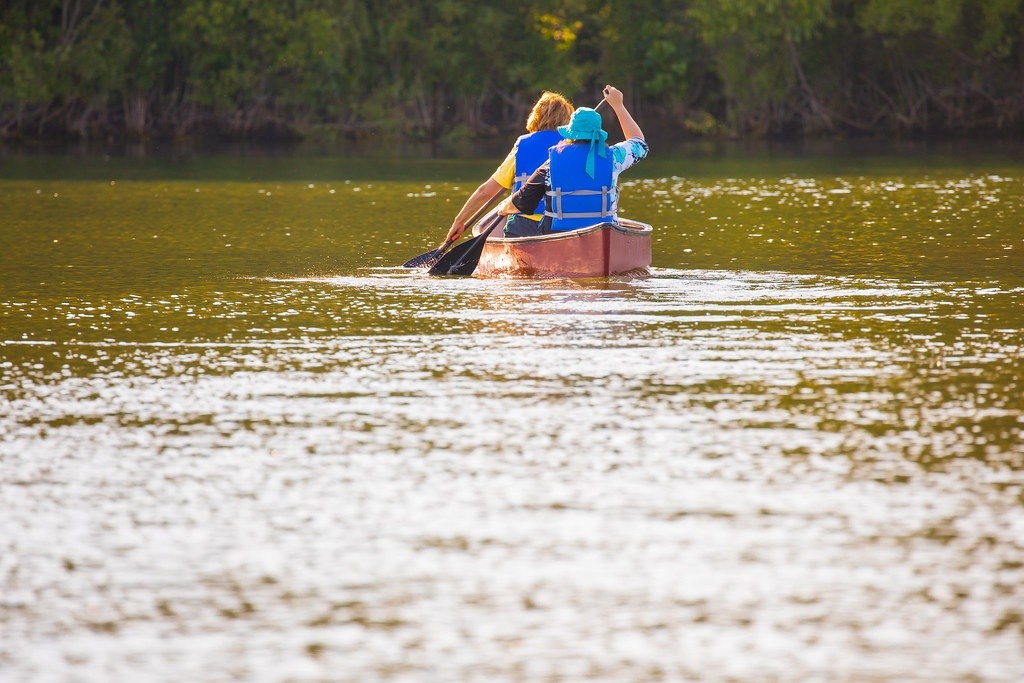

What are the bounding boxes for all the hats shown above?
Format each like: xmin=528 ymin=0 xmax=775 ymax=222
xmin=557 ymin=106 xmax=608 ymax=141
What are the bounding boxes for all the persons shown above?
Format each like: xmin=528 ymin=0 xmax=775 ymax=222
xmin=443 ymin=90 xmax=575 ymax=242
xmin=497 ymin=85 xmax=650 ymax=237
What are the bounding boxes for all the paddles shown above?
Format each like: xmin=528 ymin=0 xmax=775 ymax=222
xmin=428 ymin=87 xmax=610 ymax=276
xmin=402 ymin=177 xmax=516 ymax=268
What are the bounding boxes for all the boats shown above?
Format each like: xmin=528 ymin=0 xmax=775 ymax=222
xmin=470 ymin=193 xmax=653 ymax=278
